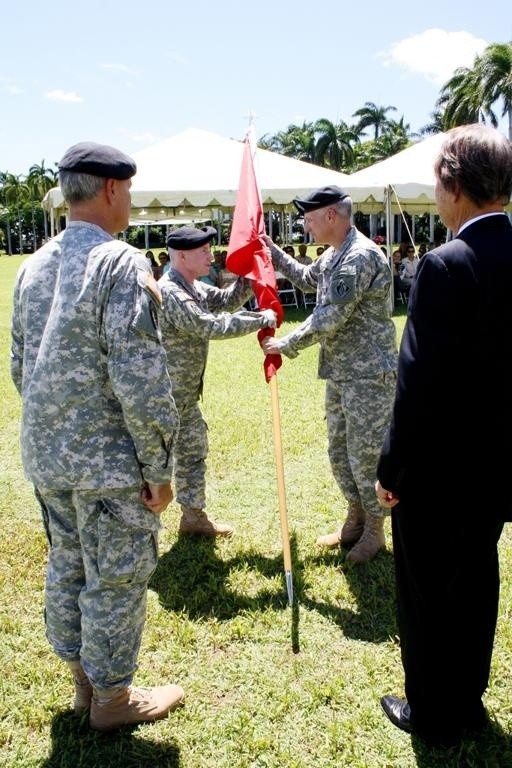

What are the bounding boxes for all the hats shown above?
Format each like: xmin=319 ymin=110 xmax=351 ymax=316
xmin=166 ymin=226 xmax=218 ymax=251
xmin=58 ymin=141 xmax=137 ymax=181
xmin=292 ymin=185 xmax=349 ymax=216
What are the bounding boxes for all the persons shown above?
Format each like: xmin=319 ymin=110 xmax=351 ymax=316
xmin=197 ymin=251 xmax=255 ymax=312
xmin=156 ymin=226 xmax=278 ymax=537
xmin=258 ymin=184 xmax=398 ymax=564
xmin=383 ymin=124 xmax=511 ymax=752
xmin=11 ymin=140 xmax=186 ymax=728
xmin=381 ymin=241 xmax=441 ymax=307
xmin=146 ymin=250 xmax=171 ymax=281
xmin=272 ymin=244 xmax=331 ymax=308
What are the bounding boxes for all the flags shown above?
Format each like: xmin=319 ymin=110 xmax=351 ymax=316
xmin=225 ymin=132 xmax=284 ymax=382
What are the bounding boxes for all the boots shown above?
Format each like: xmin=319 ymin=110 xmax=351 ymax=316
xmin=346 ymin=513 xmax=386 ymax=564
xmin=75 ymin=679 xmax=184 ymax=730
xmin=180 ymin=506 xmax=233 ymax=535
xmin=316 ymin=507 xmax=367 ymax=548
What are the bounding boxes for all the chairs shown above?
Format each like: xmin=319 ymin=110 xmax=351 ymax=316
xmin=404 ymin=293 xmax=409 ymax=303
xmin=302 ymin=291 xmax=317 ymax=309
xmin=274 ymin=271 xmax=299 ymax=309
xmin=397 ymin=293 xmax=404 ymax=305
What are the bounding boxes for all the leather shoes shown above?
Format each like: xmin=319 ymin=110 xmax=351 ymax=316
xmin=381 ymin=695 xmax=462 ymax=755
